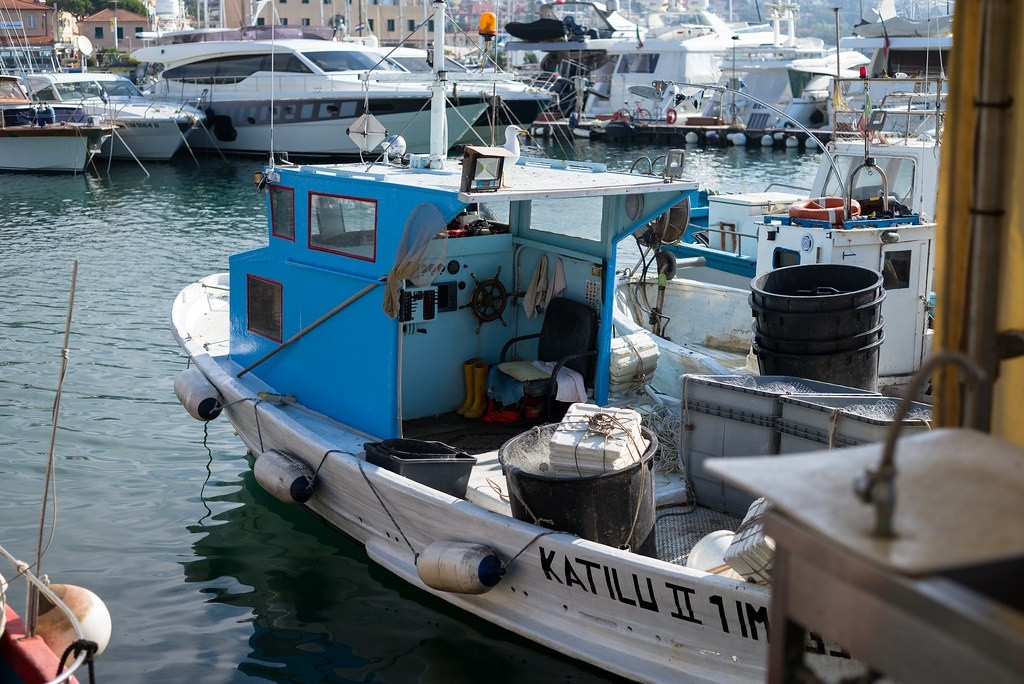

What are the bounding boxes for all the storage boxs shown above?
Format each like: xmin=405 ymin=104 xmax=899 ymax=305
xmin=676 ymin=371 xmax=932 ymax=512
xmin=365 ymin=440 xmax=478 ymax=503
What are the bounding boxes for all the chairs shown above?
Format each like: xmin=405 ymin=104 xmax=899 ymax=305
xmin=491 ymin=296 xmax=599 ymax=429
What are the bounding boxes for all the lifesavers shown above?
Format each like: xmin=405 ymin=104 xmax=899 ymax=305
xmin=788 ymin=196 xmax=862 ymax=225
xmin=665 ymin=109 xmax=678 ymax=124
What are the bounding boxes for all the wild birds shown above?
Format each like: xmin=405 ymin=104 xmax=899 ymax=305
xmin=458 ymin=124 xmax=528 ymax=188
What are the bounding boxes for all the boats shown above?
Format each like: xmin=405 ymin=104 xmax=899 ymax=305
xmin=169 ymin=1 xmax=1000 ymax=684
xmin=0 ymin=1 xmax=958 ymax=451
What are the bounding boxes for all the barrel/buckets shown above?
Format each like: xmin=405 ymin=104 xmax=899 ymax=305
xmin=499 ymin=425 xmax=658 ymax=557
xmin=747 ymin=264 xmax=886 ymax=397
